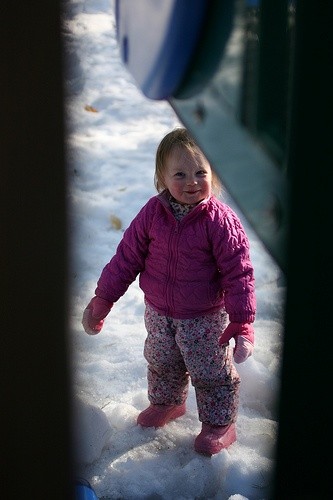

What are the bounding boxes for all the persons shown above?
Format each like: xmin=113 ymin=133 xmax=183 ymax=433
xmin=81 ymin=127 xmax=257 ymax=457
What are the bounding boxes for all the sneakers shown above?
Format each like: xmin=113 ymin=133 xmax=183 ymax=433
xmin=192 ymin=420 xmax=239 ymax=453
xmin=136 ymin=403 xmax=186 ymax=428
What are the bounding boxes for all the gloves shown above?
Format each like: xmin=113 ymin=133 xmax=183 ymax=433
xmin=81 ymin=296 xmax=113 ymax=336
xmin=220 ymin=321 xmax=256 ymax=363
